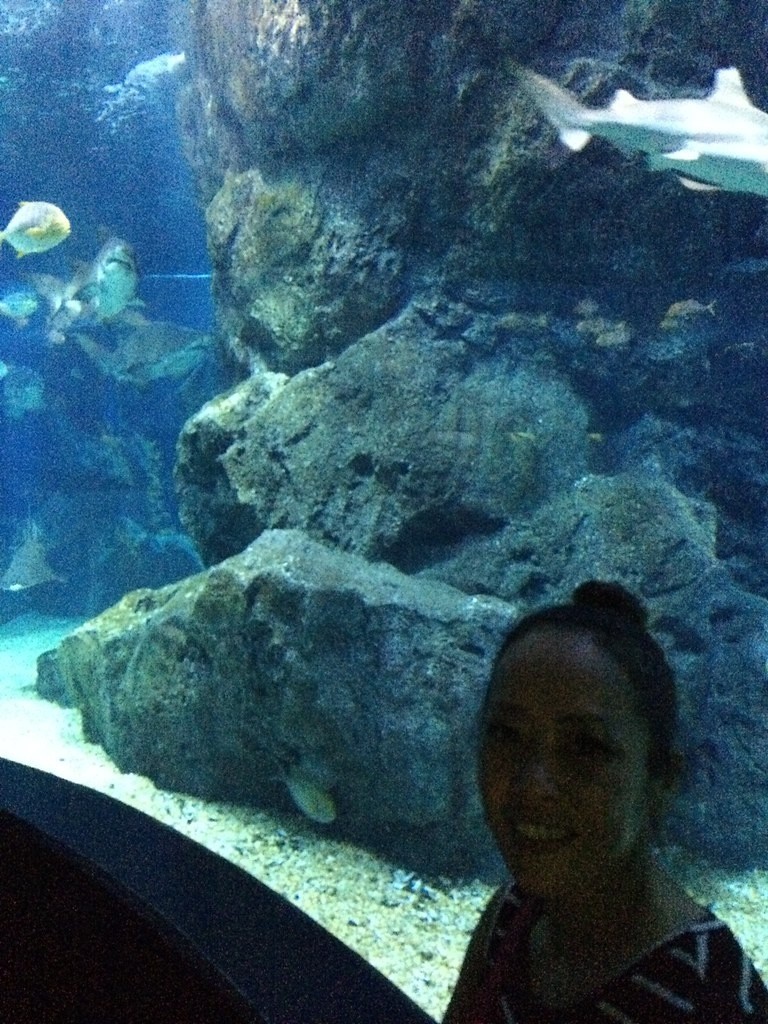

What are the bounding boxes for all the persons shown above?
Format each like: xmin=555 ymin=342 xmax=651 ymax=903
xmin=431 ymin=577 xmax=768 ymax=1024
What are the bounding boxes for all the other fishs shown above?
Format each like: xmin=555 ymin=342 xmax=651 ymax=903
xmin=0 ymin=200 xmax=72 ymax=261
xmin=1 ymin=233 xmax=219 ymax=592
